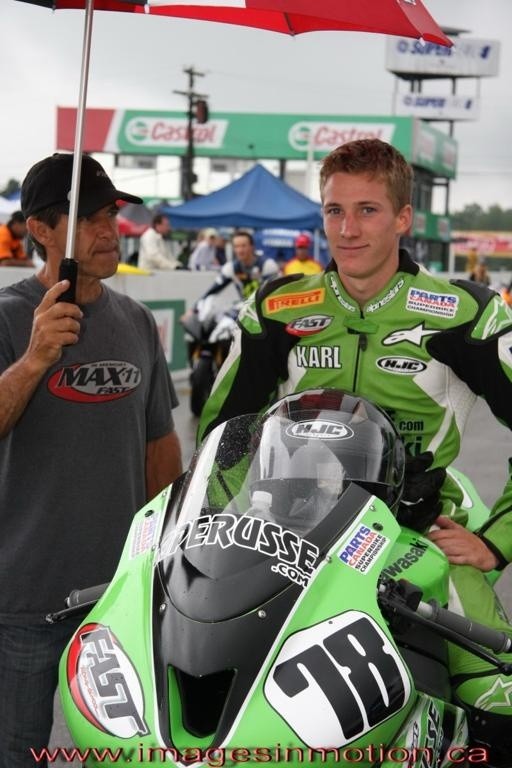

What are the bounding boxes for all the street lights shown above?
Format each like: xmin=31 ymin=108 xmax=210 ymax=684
xmin=178 ymin=100 xmax=211 ymax=202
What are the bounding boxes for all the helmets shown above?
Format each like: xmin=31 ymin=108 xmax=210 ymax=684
xmin=246 ymin=389 xmax=407 ymax=537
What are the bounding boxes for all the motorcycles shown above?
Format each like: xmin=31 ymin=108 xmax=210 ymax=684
xmin=38 ymin=387 xmax=493 ymax=767
xmin=179 ymin=312 xmax=234 ymax=415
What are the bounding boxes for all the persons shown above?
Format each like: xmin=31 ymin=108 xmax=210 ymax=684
xmin=1 ymin=210 xmax=512 ymax=322
xmin=190 ymin=133 xmax=512 ymax=762
xmin=1 ymin=152 xmax=183 ymax=768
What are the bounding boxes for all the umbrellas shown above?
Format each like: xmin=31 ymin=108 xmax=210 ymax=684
xmin=10 ymin=0 xmax=452 ymax=304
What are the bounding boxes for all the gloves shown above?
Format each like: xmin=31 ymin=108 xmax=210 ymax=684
xmin=396 ymin=451 xmax=446 ymax=535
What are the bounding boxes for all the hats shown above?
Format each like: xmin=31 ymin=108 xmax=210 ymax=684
xmin=20 ymin=153 xmax=144 ymax=220
xmin=294 ymin=236 xmax=311 ymax=249
